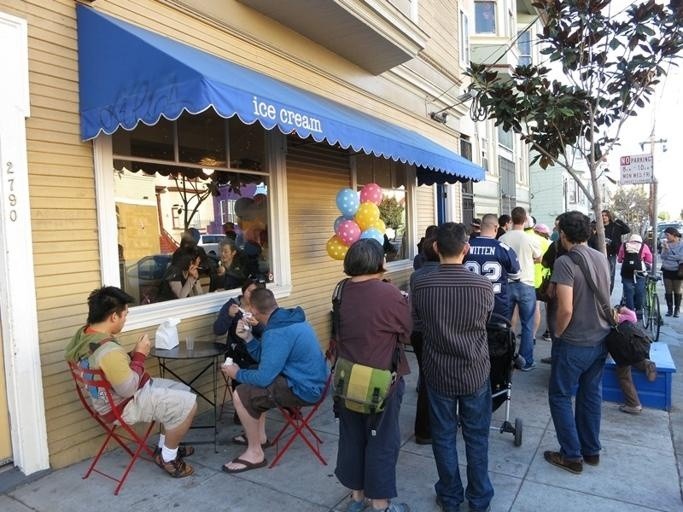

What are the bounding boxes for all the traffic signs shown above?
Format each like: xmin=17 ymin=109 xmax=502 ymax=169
xmin=619 ymin=154 xmax=654 ymax=185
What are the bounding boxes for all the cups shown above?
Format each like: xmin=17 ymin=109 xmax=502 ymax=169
xmin=185 ymin=336 xmax=194 ymax=350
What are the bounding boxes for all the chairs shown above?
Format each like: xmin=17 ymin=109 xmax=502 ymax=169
xmin=218 ymin=361 xmax=233 ymax=421
xmin=265 ymin=338 xmax=340 ymax=468
xmin=68 ymin=360 xmax=156 ymax=496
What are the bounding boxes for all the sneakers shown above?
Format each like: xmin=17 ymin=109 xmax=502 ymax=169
xmin=544 ymin=450 xmax=583 ymax=473
xmin=620 ymin=405 xmax=643 ymax=414
xmin=521 ymin=360 xmax=537 ymax=371
xmin=345 ymin=499 xmax=370 ymax=512
xmin=644 ymin=361 xmax=656 ymax=382
xmin=153 ymin=445 xmax=195 ymax=458
xmin=154 ymin=454 xmax=193 ymax=477
xmin=583 ymin=454 xmax=600 ymax=466
xmin=365 ymin=501 xmax=410 ymax=511
xmin=542 ymin=329 xmax=553 ymax=342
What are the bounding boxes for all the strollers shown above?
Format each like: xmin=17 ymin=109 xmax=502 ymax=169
xmin=456 ymin=311 xmax=522 ymax=446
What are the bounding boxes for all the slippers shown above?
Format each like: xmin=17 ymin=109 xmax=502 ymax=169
xmin=234 ymin=432 xmax=270 ymax=448
xmin=223 ymin=455 xmax=267 ymax=473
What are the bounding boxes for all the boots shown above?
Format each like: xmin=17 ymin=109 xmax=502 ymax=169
xmin=673 ymin=293 xmax=683 ymax=318
xmin=664 ymin=293 xmax=674 ymax=316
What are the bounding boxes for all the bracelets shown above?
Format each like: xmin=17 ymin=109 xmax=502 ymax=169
xmin=554 ymin=333 xmax=562 ymax=338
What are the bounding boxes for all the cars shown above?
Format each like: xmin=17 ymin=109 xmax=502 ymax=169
xmin=656 ymin=221 xmax=683 ymax=252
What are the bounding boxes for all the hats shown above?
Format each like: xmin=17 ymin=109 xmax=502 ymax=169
xmin=534 ymin=223 xmax=548 ymax=234
xmin=523 ymin=217 xmax=536 ymax=229
xmin=628 ymin=234 xmax=642 ymax=243
xmin=470 ymin=218 xmax=481 ymax=227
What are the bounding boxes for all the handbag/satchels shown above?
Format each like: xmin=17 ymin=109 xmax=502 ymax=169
xmin=329 ymin=358 xmax=392 ymax=415
xmin=605 ymin=320 xmax=652 ymax=367
xmin=537 ymin=276 xmax=554 ymax=302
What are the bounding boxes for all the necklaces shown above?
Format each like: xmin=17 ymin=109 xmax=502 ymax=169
xmin=181 ymin=268 xmax=191 ymax=278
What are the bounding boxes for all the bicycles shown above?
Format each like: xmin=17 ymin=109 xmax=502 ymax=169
xmin=633 ymin=268 xmax=664 ymax=342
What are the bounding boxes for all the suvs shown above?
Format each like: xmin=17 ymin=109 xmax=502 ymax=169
xmin=196 ymin=234 xmax=227 ymax=256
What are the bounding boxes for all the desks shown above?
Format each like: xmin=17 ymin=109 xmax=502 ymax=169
xmin=150 ymin=340 xmax=230 ymax=453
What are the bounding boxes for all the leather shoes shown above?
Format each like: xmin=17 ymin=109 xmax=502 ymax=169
xmin=541 ymin=357 xmax=553 ymax=363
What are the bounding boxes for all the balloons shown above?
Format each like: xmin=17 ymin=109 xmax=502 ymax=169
xmin=233 ymin=193 xmax=268 ymax=255
xmin=327 ymin=182 xmax=387 ymax=261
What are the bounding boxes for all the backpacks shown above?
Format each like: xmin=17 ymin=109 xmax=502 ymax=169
xmin=621 ymin=243 xmax=645 ymax=278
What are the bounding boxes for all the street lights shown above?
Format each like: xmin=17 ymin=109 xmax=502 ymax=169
xmin=640 ymin=127 xmax=667 ymax=221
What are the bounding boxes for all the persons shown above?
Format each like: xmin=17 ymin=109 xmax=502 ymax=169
xmin=222 ymin=288 xmax=330 ymax=473
xmin=209 ymin=238 xmax=259 ymax=291
xmin=329 ymin=238 xmax=411 ymax=511
xmin=65 ymin=287 xmax=198 ymax=477
xmin=152 ymin=247 xmax=203 ymax=302
xmin=409 ymin=225 xmax=496 ymax=512
xmin=467 ymin=206 xmax=653 ymax=475
xmin=213 ymin=279 xmax=302 ymax=425
xmin=414 ymin=226 xmax=439 ymax=271
xmin=660 ymin=226 xmax=682 ymax=318
